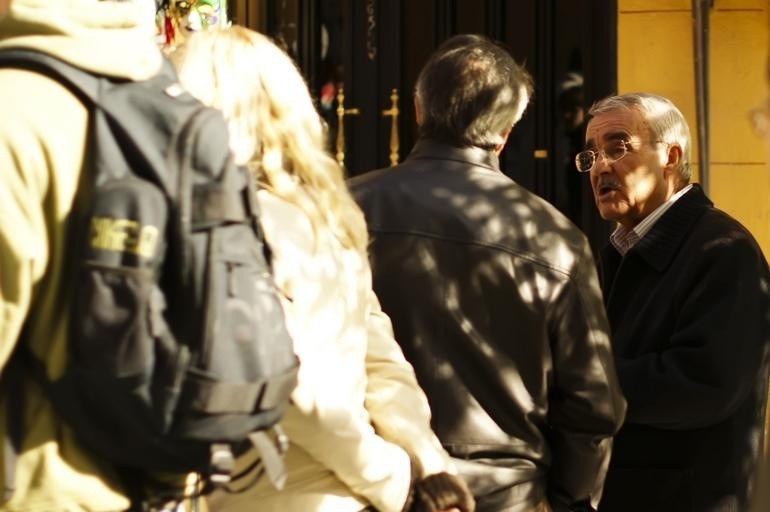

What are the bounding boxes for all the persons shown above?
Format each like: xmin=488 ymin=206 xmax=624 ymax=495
xmin=584 ymin=91 xmax=770 ymax=512
xmin=0 ymin=0 xmax=215 ymax=512
xmin=165 ymin=23 xmax=477 ymax=512
xmin=343 ymin=33 xmax=629 ymax=512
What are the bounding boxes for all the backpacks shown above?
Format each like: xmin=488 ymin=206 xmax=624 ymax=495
xmin=1 ymin=47 xmax=300 ymax=500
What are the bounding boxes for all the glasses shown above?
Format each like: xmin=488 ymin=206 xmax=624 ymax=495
xmin=575 ymin=141 xmax=661 ymax=173
